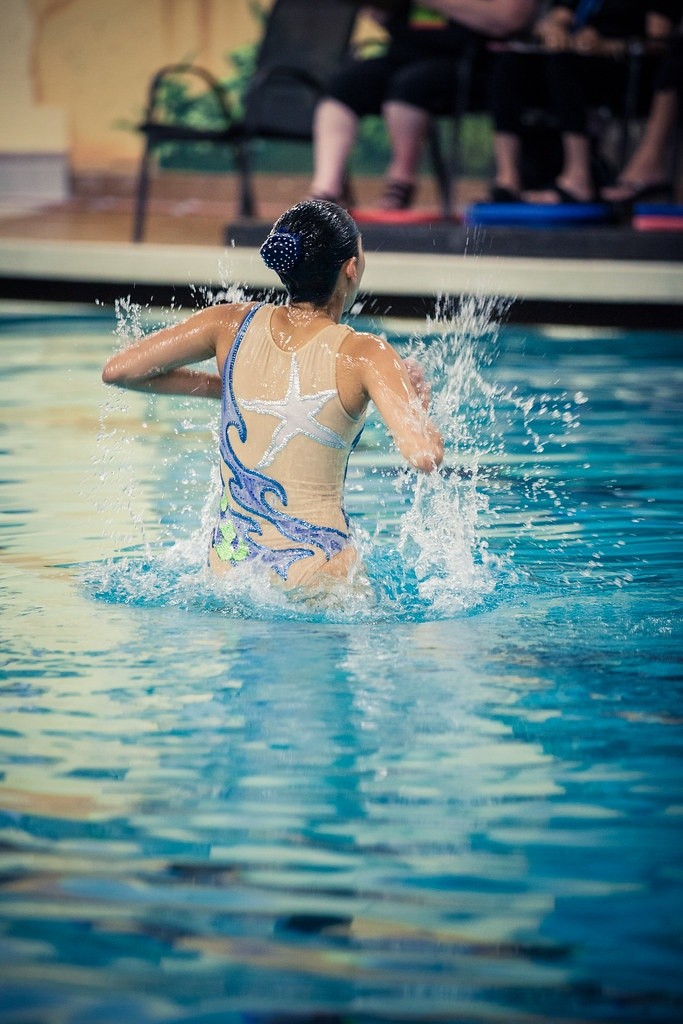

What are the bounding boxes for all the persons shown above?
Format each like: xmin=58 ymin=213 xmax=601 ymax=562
xmin=102 ymin=199 xmax=442 ymax=598
xmin=304 ymin=0 xmax=683 ymax=210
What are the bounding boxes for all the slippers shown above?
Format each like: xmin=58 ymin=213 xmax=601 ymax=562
xmin=312 ymin=193 xmax=357 ymax=211
xmin=376 ymin=175 xmax=420 ymax=209
xmin=532 ymin=179 xmax=588 ymax=203
xmin=604 ymin=179 xmax=665 ymax=207
xmin=472 ymin=183 xmax=522 ymax=206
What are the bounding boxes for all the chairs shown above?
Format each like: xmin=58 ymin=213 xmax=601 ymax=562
xmin=131 ymin=5 xmax=674 ymax=238
